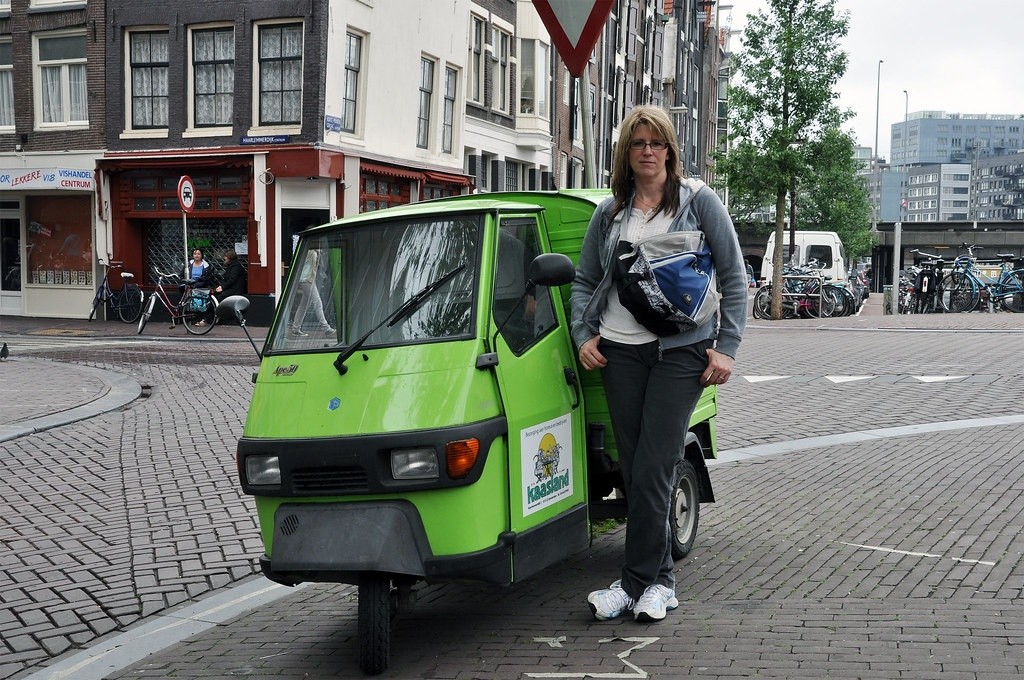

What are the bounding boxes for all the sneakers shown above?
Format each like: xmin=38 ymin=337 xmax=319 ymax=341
xmin=633 ymin=583 xmax=680 ymax=621
xmin=325 ymin=329 xmax=336 ymax=338
xmin=587 ymin=579 xmax=635 ymax=621
xmin=292 ymin=330 xmax=308 ymax=336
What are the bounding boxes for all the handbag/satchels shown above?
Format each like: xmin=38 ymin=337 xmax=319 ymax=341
xmin=188 ymin=288 xmax=210 ymax=313
xmin=614 ymin=228 xmax=719 ymax=334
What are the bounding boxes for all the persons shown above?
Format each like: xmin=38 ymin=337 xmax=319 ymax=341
xmin=525 ymin=225 xmax=555 ymax=337
xmin=570 ymin=105 xmax=748 ymax=625
xmin=178 ymin=248 xmax=214 ymax=325
xmin=195 ymin=248 xmax=258 ymax=326
xmin=291 ymin=248 xmax=337 ymax=338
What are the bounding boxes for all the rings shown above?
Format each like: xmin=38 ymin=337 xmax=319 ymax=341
xmin=719 ymin=376 xmax=725 ymax=380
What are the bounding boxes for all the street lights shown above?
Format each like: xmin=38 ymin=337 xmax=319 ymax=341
xmin=869 ymin=58 xmax=883 ymax=231
xmin=900 ymin=90 xmax=908 ymax=222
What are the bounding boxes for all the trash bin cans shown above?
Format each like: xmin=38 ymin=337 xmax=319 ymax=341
xmin=244 ymin=292 xmax=275 ymax=327
xmin=883 ymin=285 xmax=894 ymax=315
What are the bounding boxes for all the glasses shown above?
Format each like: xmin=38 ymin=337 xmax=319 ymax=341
xmin=629 ymin=139 xmax=669 ymax=150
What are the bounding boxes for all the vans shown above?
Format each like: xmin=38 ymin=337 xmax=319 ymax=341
xmin=758 ymin=230 xmax=851 ymax=290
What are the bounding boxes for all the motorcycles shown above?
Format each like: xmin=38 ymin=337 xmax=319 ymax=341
xmin=216 ymin=188 xmax=720 ymax=679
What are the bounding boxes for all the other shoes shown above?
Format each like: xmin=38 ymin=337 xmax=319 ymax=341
xmin=196 ymin=320 xmax=210 ymax=326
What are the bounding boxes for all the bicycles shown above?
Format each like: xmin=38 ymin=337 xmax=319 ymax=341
xmin=137 ymin=264 xmax=220 ymax=336
xmin=752 ymin=257 xmax=870 ymax=322
xmin=88 ymin=258 xmax=145 ymax=324
xmin=898 ymin=242 xmax=1024 ymax=315
